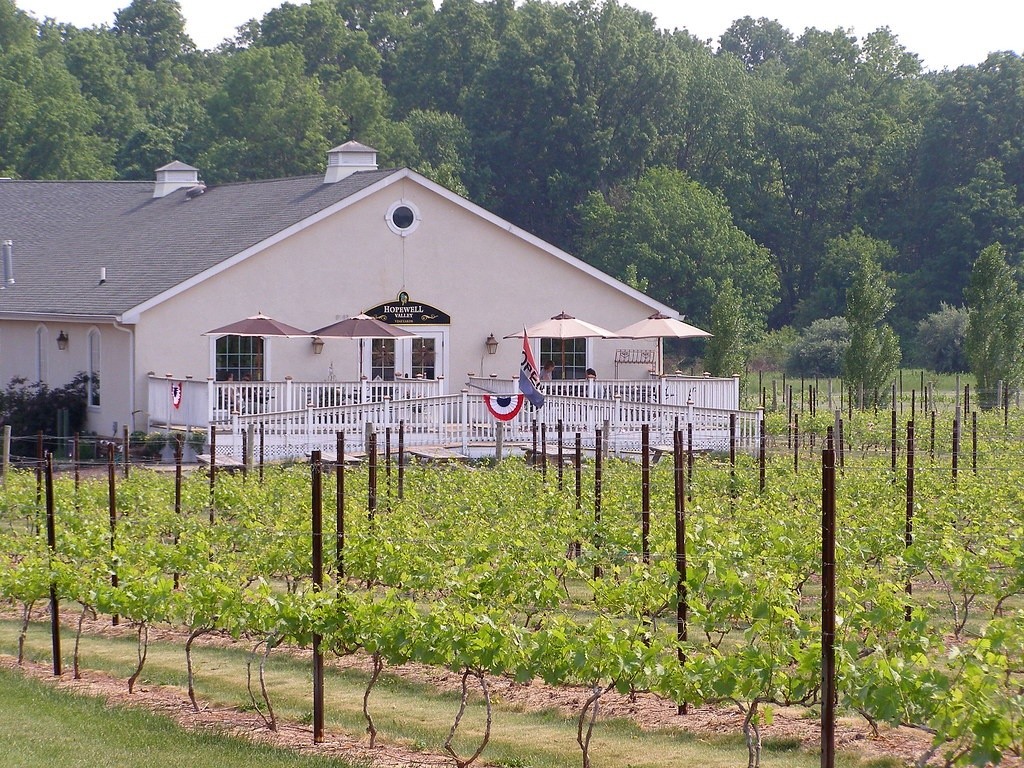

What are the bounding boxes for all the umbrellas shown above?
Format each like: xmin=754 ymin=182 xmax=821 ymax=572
xmin=201 ymin=311 xmax=421 ymax=339
xmin=503 ymin=311 xmax=714 ymax=339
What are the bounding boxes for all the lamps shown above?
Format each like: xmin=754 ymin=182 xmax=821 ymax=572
xmin=56 ymin=330 xmax=68 ymax=351
xmin=310 ymin=337 xmax=325 ymax=354
xmin=485 ymin=332 xmax=499 ymax=354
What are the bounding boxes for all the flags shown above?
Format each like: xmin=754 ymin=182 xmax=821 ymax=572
xmin=520 ymin=327 xmax=546 ymax=409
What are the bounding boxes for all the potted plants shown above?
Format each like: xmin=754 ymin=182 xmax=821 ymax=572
xmin=187 ymin=430 xmax=206 ymax=464
xmin=143 ymin=431 xmax=167 ymax=464
xmin=166 ymin=430 xmax=186 ymax=462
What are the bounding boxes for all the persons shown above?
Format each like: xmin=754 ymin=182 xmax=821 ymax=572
xmin=540 ymin=361 xmax=597 ymax=380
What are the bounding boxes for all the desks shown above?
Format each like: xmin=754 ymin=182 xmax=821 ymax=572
xmin=649 ymin=444 xmax=714 ymax=463
xmin=519 ymin=445 xmax=584 ymax=468
xmin=305 ymin=450 xmax=362 ymax=471
xmin=195 ymin=454 xmax=247 ymax=477
xmin=409 ymin=446 xmax=469 ymax=464
